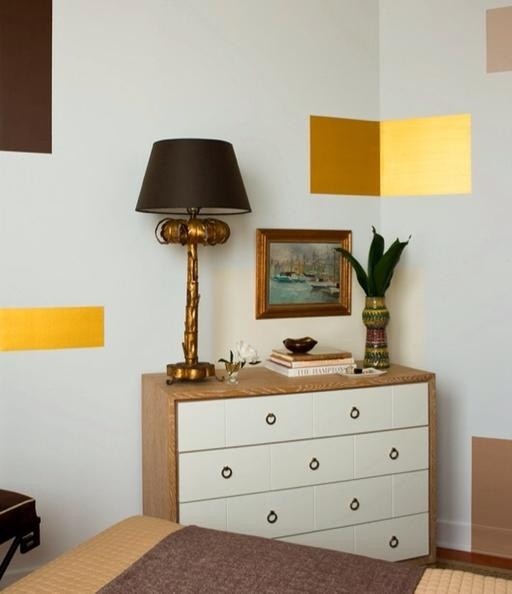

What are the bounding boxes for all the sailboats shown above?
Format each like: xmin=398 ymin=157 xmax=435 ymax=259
xmin=272 ymin=244 xmax=340 ymax=297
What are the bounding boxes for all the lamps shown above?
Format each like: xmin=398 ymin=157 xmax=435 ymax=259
xmin=137 ymin=139 xmax=252 ymax=384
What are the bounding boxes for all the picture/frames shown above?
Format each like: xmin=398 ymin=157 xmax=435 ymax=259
xmin=255 ymin=228 xmax=354 ymax=322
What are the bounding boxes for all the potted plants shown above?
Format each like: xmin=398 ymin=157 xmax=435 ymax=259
xmin=332 ymin=225 xmax=412 ymax=370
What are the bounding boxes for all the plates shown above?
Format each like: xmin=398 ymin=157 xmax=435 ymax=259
xmin=341 ymin=368 xmax=387 ymax=379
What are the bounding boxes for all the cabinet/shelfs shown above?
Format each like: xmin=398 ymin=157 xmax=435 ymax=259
xmin=141 ymin=360 xmax=438 ymax=567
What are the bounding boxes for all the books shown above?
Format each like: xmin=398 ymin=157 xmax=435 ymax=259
xmin=264 ymin=359 xmax=355 ymax=377
xmin=268 ymin=354 xmax=354 ymax=368
xmin=270 ymin=348 xmax=353 ymax=360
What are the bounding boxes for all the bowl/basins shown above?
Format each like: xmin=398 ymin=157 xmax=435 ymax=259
xmin=284 ymin=337 xmax=318 ymax=353
